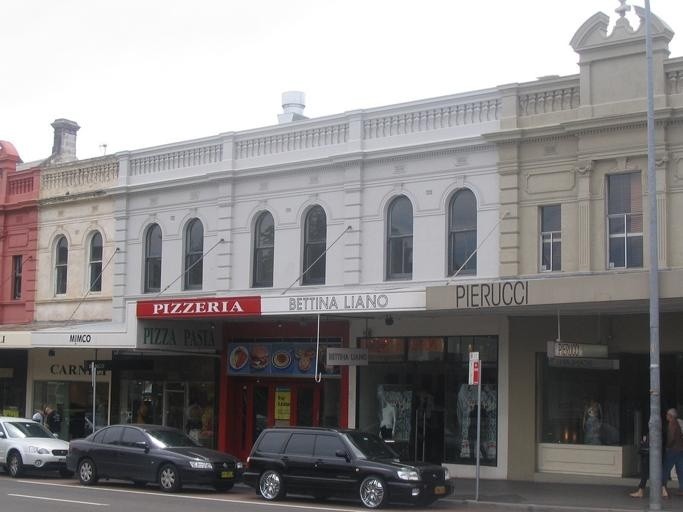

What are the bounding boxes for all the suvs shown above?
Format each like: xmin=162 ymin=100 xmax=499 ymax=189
xmin=242 ymin=425 xmax=454 ymax=509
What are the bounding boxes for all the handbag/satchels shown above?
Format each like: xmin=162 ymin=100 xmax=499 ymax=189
xmin=638 ymin=447 xmax=649 ymax=454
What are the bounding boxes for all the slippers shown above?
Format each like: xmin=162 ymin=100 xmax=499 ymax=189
xmin=629 ymin=493 xmax=643 ymax=498
xmin=662 ymin=496 xmax=669 ymax=499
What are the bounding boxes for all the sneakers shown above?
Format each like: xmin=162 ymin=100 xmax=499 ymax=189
xmin=674 ymin=491 xmax=683 ymax=495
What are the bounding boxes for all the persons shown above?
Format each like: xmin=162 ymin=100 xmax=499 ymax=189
xmin=662 ymin=408 xmax=683 ymax=496
xmin=45 ymin=407 xmax=60 ymax=433
xmin=379 ymin=403 xmax=396 ymax=440
xmin=582 ymin=399 xmax=603 ymax=443
xmin=629 ymin=430 xmax=668 ymax=498
xmin=467 ymin=405 xmax=490 ymax=463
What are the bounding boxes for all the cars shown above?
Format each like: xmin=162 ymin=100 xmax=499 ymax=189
xmin=66 ymin=424 xmax=242 ymax=493
xmin=0 ymin=416 xmax=69 ymax=478
